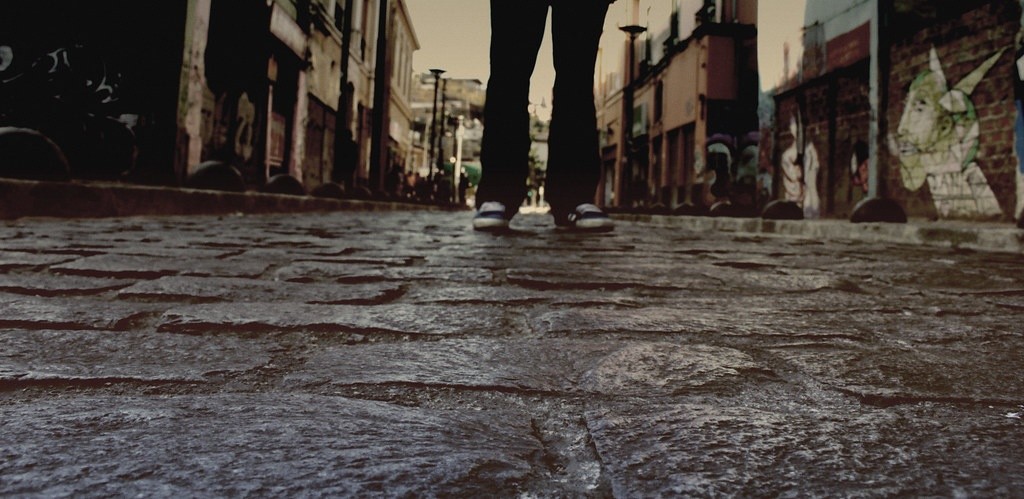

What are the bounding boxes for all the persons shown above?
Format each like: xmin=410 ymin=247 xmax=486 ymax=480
xmin=384 ymin=164 xmax=468 ymax=206
xmin=472 ymin=0 xmax=620 ymax=233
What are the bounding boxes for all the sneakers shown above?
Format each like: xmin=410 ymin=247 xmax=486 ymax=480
xmin=554 ymin=203 xmax=614 ymax=230
xmin=472 ymin=202 xmax=509 ymax=231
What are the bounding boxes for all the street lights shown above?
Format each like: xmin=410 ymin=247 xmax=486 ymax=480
xmin=617 ymin=25 xmax=648 ymax=212
xmin=424 ymin=68 xmax=445 ymax=210
